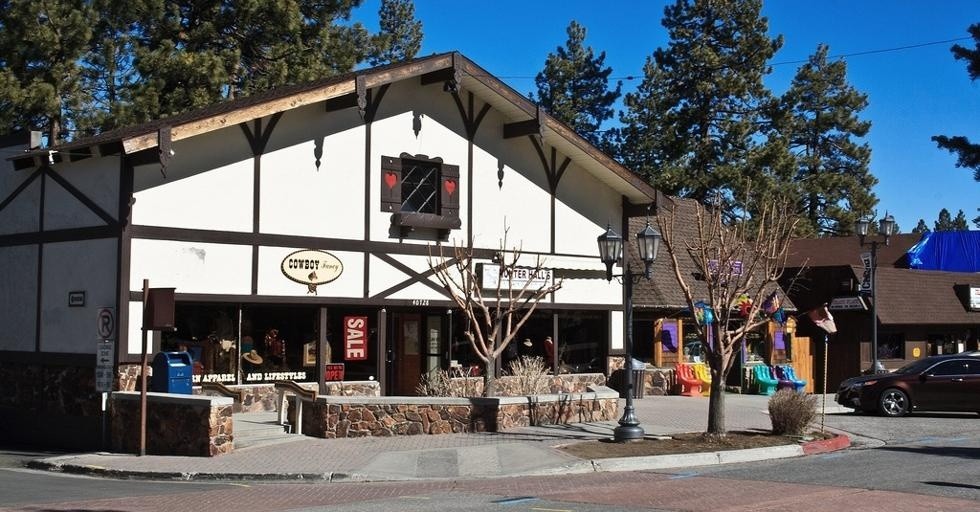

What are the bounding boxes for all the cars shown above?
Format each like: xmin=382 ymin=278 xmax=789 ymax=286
xmin=834 ymin=355 xmax=980 ymax=417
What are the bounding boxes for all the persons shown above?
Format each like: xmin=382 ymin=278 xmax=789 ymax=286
xmin=544 ymin=336 xmax=554 ymax=366
xmin=523 ymin=338 xmax=534 ymax=356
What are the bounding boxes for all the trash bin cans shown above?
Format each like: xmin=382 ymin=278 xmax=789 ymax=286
xmin=152 ymin=351 xmax=193 ymax=394
xmin=618 ymin=367 xmax=644 ymax=399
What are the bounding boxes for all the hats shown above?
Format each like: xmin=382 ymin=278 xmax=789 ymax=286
xmin=242 ymin=349 xmax=264 ymax=365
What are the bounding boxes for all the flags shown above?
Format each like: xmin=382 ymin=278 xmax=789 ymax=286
xmin=686 ymin=301 xmax=714 ymax=325
xmin=809 ymin=306 xmax=838 ymax=335
xmin=732 ymin=292 xmax=758 ymax=319
xmin=761 ymin=292 xmax=786 ymax=324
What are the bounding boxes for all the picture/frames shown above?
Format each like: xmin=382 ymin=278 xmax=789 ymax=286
xmin=302 ymin=339 xmax=333 ymax=368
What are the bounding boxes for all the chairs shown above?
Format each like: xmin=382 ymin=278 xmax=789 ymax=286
xmin=753 ymin=364 xmax=777 ymax=397
xmin=674 ymin=363 xmax=703 ymax=399
xmin=768 ymin=365 xmax=792 ymax=395
xmin=692 ymin=362 xmax=711 ymax=397
xmin=782 ymin=363 xmax=807 ymax=399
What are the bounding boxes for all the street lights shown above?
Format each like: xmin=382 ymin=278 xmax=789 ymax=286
xmin=854 ymin=208 xmax=895 ymax=374
xmin=597 ymin=213 xmax=662 ymax=443
